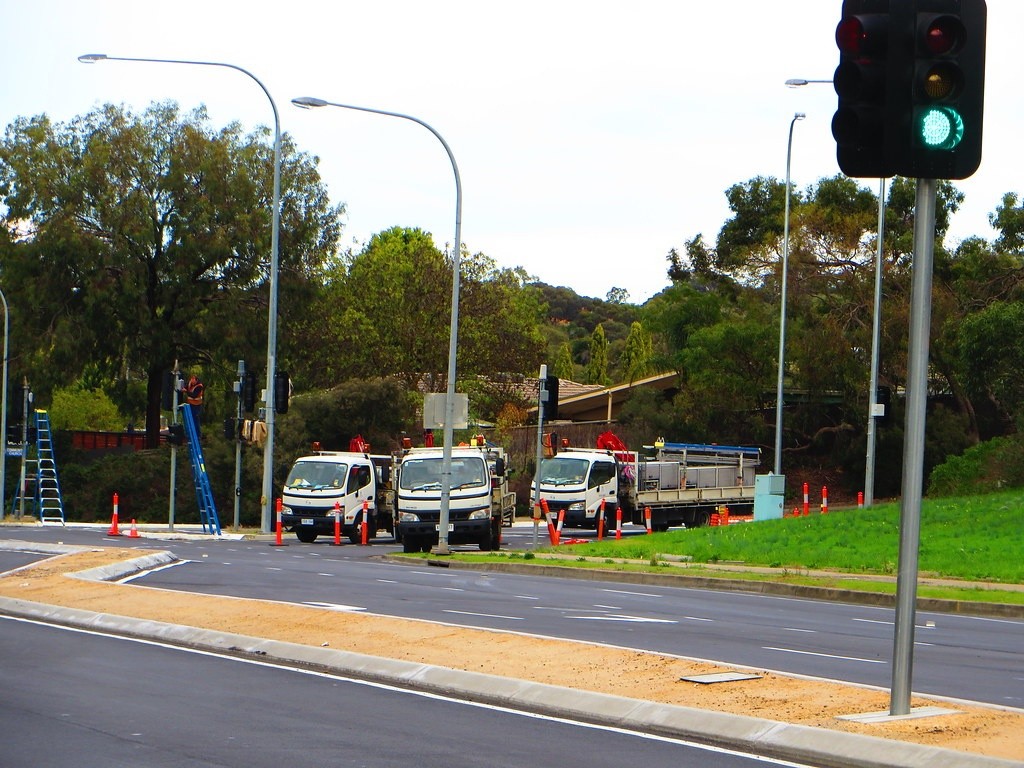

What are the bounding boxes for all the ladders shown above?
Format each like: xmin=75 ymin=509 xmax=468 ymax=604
xmin=178 ymin=403 xmax=222 ymax=536
xmin=11 ymin=409 xmax=65 ymax=526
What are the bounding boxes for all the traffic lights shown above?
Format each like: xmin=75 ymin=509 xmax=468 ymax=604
xmin=222 ymin=417 xmax=234 ymax=441
xmin=164 ymin=422 xmax=183 ymax=447
xmin=244 ymin=373 xmax=259 ymax=413
xmin=542 ymin=433 xmax=558 ymax=458
xmin=830 ymin=0 xmax=921 ymax=180
xmin=909 ymin=0 xmax=988 ymax=179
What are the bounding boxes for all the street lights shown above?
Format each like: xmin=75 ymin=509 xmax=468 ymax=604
xmin=77 ymin=53 xmax=276 ymax=533
xmin=291 ymin=97 xmax=463 ymax=571
xmin=772 ymin=111 xmax=808 ymax=476
xmin=786 ymin=79 xmax=877 ymax=508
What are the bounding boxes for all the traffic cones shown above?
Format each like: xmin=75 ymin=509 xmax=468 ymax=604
xmin=127 ymin=519 xmax=142 ymax=538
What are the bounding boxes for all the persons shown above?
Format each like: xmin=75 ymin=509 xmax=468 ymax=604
xmin=182 ymin=375 xmax=204 ymax=437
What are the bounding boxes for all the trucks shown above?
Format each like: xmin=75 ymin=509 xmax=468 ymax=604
xmin=392 ymin=433 xmax=518 ymax=554
xmin=282 ymin=441 xmax=402 ymax=544
xmin=530 ymin=437 xmax=762 ymax=537
